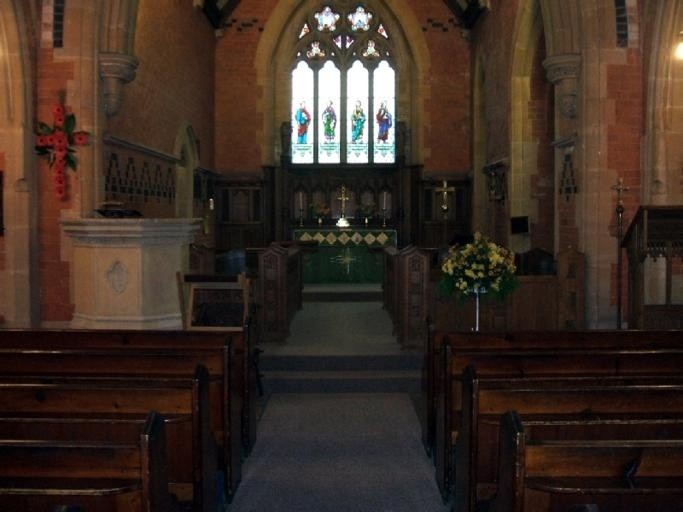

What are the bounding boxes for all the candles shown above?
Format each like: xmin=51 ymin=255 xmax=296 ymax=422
xmin=383 ymin=191 xmax=387 ymax=209
xmin=299 ymin=189 xmax=303 ymax=209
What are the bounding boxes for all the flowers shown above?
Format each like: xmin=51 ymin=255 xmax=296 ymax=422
xmin=436 ymin=230 xmax=517 ymax=305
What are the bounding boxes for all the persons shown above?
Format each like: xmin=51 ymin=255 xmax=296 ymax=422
xmin=295 ymin=101 xmax=310 ymax=144
xmin=322 ymin=100 xmax=336 ymax=144
xmin=376 ymin=103 xmax=392 ymax=144
xmin=350 ymin=100 xmax=366 ymax=143
